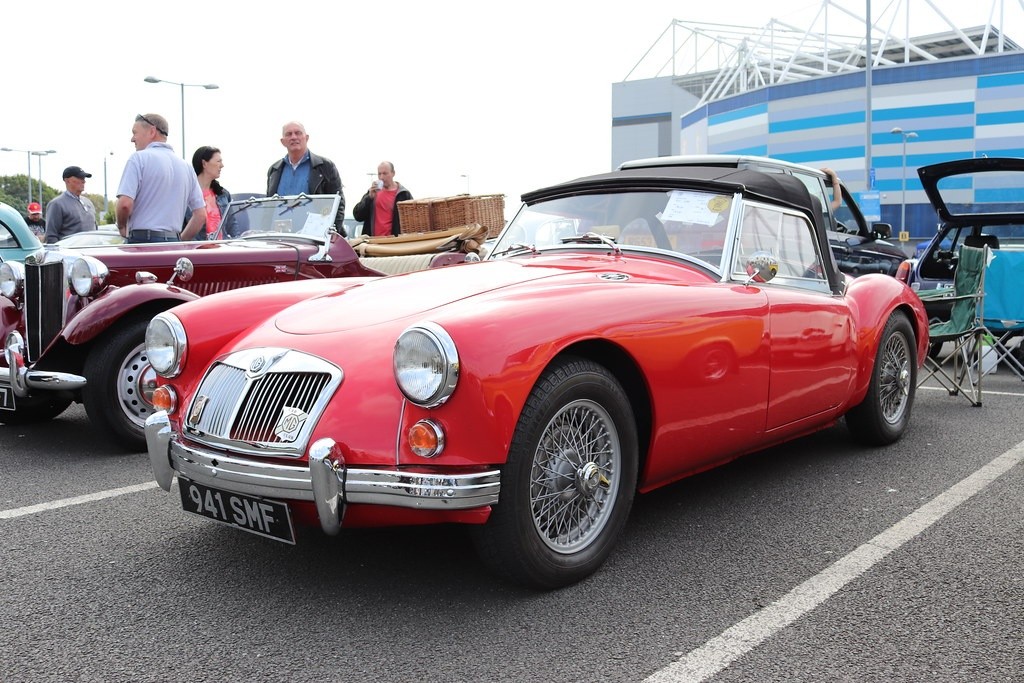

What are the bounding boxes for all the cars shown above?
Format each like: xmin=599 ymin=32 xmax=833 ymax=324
xmin=576 ymin=154 xmax=909 ymax=282
xmin=1 ymin=201 xmax=45 ymax=264
xmin=143 ymin=169 xmax=931 ymax=592
xmin=894 ymin=157 xmax=1024 ymax=361
xmin=0 ymin=191 xmax=507 ymax=447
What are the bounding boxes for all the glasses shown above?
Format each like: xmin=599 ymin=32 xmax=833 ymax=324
xmin=135 ymin=114 xmax=168 ymax=136
xmin=201 ymin=146 xmax=216 ymax=156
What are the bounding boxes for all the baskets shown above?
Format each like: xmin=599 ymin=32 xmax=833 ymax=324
xmin=396 ymin=194 xmax=471 ymax=233
xmin=469 ymin=193 xmax=506 ymax=238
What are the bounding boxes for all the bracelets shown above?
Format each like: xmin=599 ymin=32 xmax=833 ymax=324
xmin=832 ymin=181 xmax=839 ymax=185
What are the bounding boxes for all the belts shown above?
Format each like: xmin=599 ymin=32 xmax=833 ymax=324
xmin=128 ymin=231 xmax=179 ymax=239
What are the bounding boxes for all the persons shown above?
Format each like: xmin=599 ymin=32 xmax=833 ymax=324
xmin=116 ymin=114 xmax=207 ymax=244
xmin=267 ymin=122 xmax=345 ymax=228
xmin=353 ymin=161 xmax=414 ymax=240
xmin=818 ymin=166 xmax=843 ymax=212
xmin=184 ymin=145 xmax=241 ymax=242
xmin=46 ymin=166 xmax=97 ymax=245
xmin=24 ymin=201 xmax=46 ymax=245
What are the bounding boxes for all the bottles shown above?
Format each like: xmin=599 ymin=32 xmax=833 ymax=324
xmin=977 ymin=333 xmax=998 ymax=374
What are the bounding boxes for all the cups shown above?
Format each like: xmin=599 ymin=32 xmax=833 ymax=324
xmin=374 ymin=180 xmax=384 ymax=192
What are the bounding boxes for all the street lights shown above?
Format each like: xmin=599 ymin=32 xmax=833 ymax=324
xmin=31 ymin=152 xmax=47 ymax=209
xmin=143 ymin=77 xmax=220 ymax=161
xmin=104 ymin=151 xmax=114 ymax=211
xmin=0 ymin=147 xmax=56 ymax=203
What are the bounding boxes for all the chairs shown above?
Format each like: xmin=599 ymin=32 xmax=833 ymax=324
xmin=914 ymin=243 xmax=989 ymax=408
xmin=618 ymin=217 xmax=657 ymax=248
xmin=958 ymin=249 xmax=1024 ymax=386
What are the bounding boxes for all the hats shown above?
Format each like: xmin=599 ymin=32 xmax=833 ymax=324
xmin=28 ymin=202 xmax=43 ymax=214
xmin=63 ymin=166 xmax=92 ymax=181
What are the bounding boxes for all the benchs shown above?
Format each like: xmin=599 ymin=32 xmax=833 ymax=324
xmin=358 ymin=254 xmax=435 ymax=275
